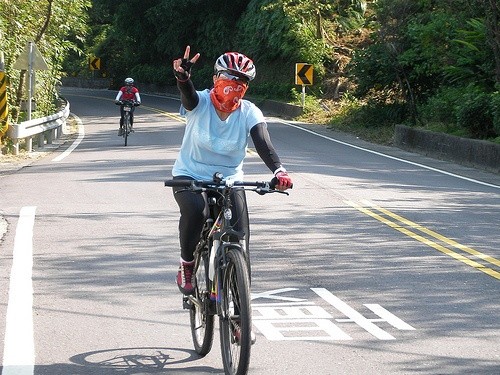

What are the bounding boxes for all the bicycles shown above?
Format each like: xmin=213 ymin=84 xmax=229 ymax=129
xmin=164 ymin=172 xmax=293 ymax=375
xmin=115 ymin=99 xmax=141 ymax=146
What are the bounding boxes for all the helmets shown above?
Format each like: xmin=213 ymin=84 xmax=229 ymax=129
xmin=214 ymin=52 xmax=256 ymax=82
xmin=124 ymin=78 xmax=134 ymax=84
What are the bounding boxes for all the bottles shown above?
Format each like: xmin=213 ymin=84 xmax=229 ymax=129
xmin=208 ymin=240 xmax=220 ymax=281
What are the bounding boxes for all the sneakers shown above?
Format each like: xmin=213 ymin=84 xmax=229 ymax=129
xmin=229 ymin=314 xmax=258 ymax=346
xmin=174 ymin=256 xmax=197 ymax=295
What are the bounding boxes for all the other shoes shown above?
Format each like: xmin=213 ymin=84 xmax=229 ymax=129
xmin=130 ymin=125 xmax=135 ymax=132
xmin=118 ymin=128 xmax=122 ymax=136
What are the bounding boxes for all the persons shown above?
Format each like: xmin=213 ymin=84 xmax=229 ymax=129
xmin=114 ymin=78 xmax=141 ymax=136
xmin=172 ymin=45 xmax=293 ymax=346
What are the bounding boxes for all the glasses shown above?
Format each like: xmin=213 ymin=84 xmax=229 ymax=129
xmin=126 ymin=84 xmax=131 ymax=87
xmin=220 ymin=71 xmax=248 ymax=84
